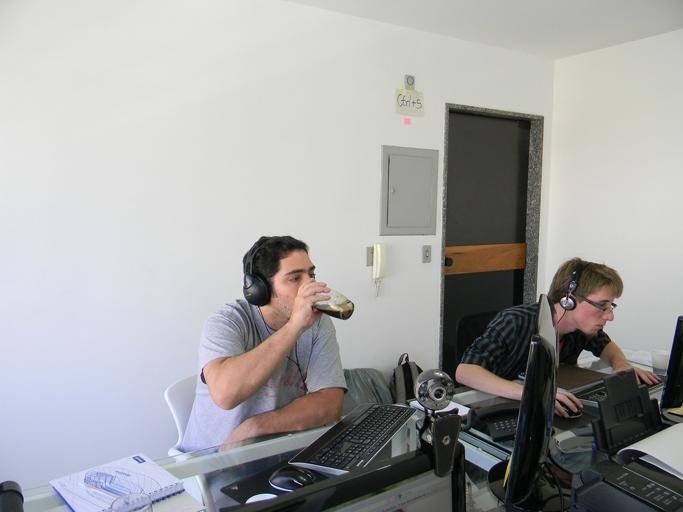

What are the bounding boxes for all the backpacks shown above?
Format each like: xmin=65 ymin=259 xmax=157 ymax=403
xmin=389 ymin=353 xmax=423 ymax=403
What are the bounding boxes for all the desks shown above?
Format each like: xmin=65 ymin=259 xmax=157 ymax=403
xmin=0 ymin=347 xmax=683 ymax=512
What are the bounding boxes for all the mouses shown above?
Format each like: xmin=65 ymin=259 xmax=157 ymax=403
xmin=559 ymin=397 xmax=583 ymax=420
xmin=270 ymin=465 xmax=315 ymax=491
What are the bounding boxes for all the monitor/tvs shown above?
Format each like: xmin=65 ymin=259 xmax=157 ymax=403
xmin=488 ymin=292 xmax=561 ymax=512
xmin=660 ymin=316 xmax=683 ymax=411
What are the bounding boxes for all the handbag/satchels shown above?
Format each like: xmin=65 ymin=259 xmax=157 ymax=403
xmin=341 ymin=367 xmax=393 ymax=415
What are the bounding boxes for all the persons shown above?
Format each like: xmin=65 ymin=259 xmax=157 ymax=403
xmin=459 ymin=257 xmax=662 ymax=420
xmin=168 ymin=236 xmax=349 ymax=454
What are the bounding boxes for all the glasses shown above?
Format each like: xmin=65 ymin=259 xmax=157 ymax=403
xmin=579 ymin=295 xmax=617 ymax=313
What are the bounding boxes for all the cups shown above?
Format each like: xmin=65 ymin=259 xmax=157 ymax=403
xmin=313 ymin=288 xmax=354 ymax=321
xmin=112 ymin=493 xmax=152 ymax=512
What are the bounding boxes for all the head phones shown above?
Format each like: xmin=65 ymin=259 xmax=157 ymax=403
xmin=241 ymin=239 xmax=271 ymax=306
xmin=559 ymin=261 xmax=583 ymax=310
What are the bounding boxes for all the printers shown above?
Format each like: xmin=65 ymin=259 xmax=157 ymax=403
xmin=576 ymin=368 xmax=683 ymax=508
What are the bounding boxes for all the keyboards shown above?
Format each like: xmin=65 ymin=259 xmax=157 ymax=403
xmin=289 ymin=403 xmax=418 ymax=477
xmin=575 ymin=375 xmax=665 ymax=405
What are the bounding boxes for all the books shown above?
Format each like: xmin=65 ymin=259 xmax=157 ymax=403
xmin=48 ymin=454 xmax=185 ymax=512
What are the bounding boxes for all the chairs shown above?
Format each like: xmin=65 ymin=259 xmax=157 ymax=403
xmin=456 ymin=311 xmax=499 ymax=367
xmin=163 ymin=374 xmax=195 ymax=456
